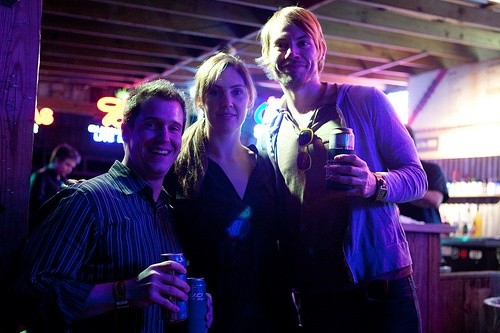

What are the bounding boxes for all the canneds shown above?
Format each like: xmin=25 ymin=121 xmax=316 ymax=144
xmin=329 ymin=127 xmax=355 ymax=150
xmin=186 ymin=277 xmax=209 ymax=333
xmin=160 ymin=253 xmax=188 ymax=321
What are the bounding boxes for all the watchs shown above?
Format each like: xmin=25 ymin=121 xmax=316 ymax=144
xmin=371 ymin=173 xmax=388 ymax=202
xmin=112 ymin=280 xmax=130 ymax=310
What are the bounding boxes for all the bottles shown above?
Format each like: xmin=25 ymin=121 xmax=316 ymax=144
xmin=470 ymin=200 xmax=483 ymax=238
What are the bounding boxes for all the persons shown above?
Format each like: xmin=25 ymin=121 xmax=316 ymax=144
xmin=391 ymin=123 xmax=449 ymax=225
xmin=18 ymin=78 xmax=215 ymax=333
xmin=250 ymin=6 xmax=429 ymax=333
xmin=61 ymin=51 xmax=309 ymax=333
xmin=27 ymin=142 xmax=82 ymax=223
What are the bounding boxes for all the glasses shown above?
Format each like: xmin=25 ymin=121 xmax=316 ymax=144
xmin=296 ymin=128 xmax=313 ymax=171
